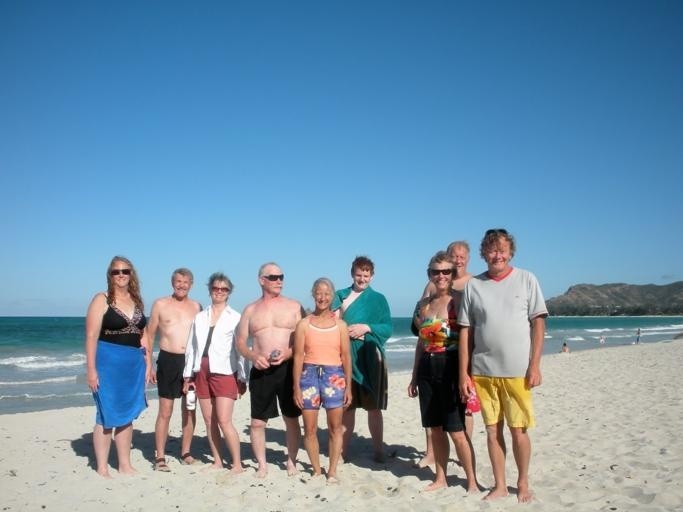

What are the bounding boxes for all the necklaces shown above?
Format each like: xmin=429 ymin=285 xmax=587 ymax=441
xmin=114 ymin=293 xmax=130 ymax=300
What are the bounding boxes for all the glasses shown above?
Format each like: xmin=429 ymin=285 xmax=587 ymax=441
xmin=430 ymin=268 xmax=454 ymax=275
xmin=109 ymin=269 xmax=133 ymax=277
xmin=263 ymin=273 xmax=283 ymax=281
xmin=210 ymin=286 xmax=230 ymax=292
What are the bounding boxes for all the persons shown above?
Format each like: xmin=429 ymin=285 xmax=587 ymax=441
xmin=411 ymin=241 xmax=478 ymax=470
xmin=233 ymin=262 xmax=308 ymax=480
xmin=599 ymin=335 xmax=605 ymax=344
xmin=562 ymin=343 xmax=569 ymax=353
xmin=408 ymin=249 xmax=486 ymax=495
xmin=455 ymin=227 xmax=548 ymax=504
xmin=84 ymin=256 xmax=153 ymax=479
xmin=141 ymin=267 xmax=205 ymax=473
xmin=635 ymin=329 xmax=641 ymax=344
xmin=290 ymin=278 xmax=356 ymax=486
xmin=330 ymin=255 xmax=394 ymax=465
xmin=181 ymin=271 xmax=248 ymax=477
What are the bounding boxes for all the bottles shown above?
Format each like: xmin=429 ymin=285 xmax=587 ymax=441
xmin=186 ymin=390 xmax=195 ymax=410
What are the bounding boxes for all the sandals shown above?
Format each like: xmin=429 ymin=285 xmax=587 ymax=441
xmin=152 ymin=457 xmax=170 ymax=473
xmin=177 ymin=448 xmax=196 ymax=465
